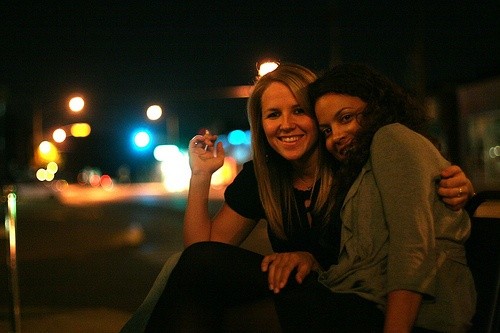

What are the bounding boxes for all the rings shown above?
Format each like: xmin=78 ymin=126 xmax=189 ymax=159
xmin=457 ymin=186 xmax=463 ymax=197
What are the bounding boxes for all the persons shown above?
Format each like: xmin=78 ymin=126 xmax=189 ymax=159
xmin=115 ymin=65 xmax=479 ymax=333
xmin=142 ymin=65 xmax=479 ymax=333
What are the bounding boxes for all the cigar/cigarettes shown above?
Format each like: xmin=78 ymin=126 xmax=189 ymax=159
xmin=205 ymin=129 xmax=210 ymax=135
